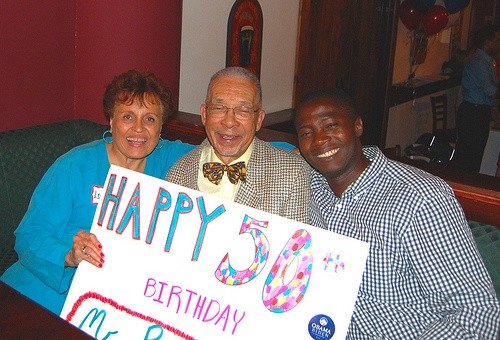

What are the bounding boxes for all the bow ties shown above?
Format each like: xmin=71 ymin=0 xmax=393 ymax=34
xmin=203 ymin=161 xmax=247 ymax=185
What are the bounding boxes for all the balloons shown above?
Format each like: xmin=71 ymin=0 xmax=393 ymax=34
xmin=424 ymin=5 xmax=449 ymax=36
xmin=444 ymin=0 xmax=470 ymax=14
xmin=412 ymin=0 xmax=436 ymax=16
xmin=399 ymin=0 xmax=424 ymax=32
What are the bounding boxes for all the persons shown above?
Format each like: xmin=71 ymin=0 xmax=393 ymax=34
xmin=0 ymin=68 xmax=300 ymax=317
xmin=456 ymin=23 xmax=500 ymax=187
xmin=164 ymin=69 xmax=309 ymax=227
xmin=289 ymin=89 xmax=500 ymax=340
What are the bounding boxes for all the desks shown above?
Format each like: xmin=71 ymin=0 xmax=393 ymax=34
xmin=479 ymin=122 xmax=500 ymax=176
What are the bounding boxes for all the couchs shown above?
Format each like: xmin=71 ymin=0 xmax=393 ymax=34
xmin=0 ymin=119 xmax=500 ymax=340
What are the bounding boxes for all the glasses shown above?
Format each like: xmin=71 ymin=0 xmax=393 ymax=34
xmin=206 ymin=103 xmax=260 ymax=120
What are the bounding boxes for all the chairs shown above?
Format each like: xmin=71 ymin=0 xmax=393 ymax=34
xmin=427 ymin=94 xmax=456 ymax=160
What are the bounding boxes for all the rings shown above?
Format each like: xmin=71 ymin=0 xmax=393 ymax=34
xmin=81 ymin=245 xmax=87 ymax=255
